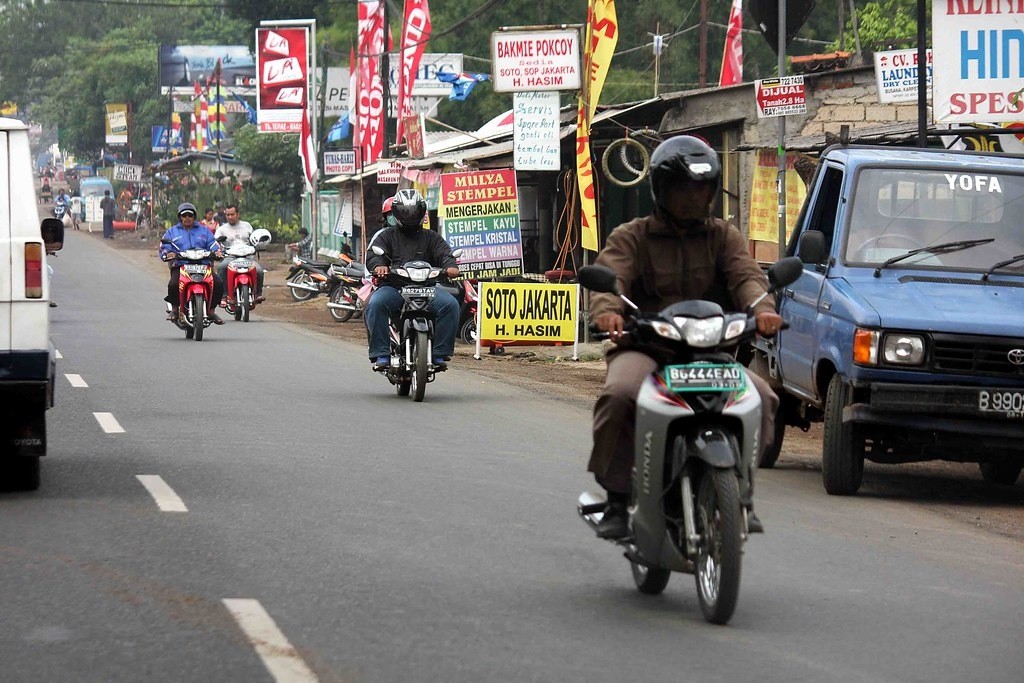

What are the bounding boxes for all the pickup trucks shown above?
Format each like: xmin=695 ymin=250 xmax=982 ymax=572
xmin=756 ymin=143 xmax=1024 ymax=496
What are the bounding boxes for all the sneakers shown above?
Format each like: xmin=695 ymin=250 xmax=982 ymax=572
xmin=166 ymin=307 xmax=177 ymax=320
xmin=376 ymin=353 xmax=392 ymax=369
xmin=595 ymin=502 xmax=631 ymax=540
xmin=432 ymin=354 xmax=446 ymax=371
xmin=208 ymin=313 xmax=224 ymax=323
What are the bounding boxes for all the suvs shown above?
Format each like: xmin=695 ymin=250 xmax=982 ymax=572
xmin=0 ymin=116 xmax=65 ymax=488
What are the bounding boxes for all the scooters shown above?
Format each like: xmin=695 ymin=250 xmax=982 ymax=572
xmin=54 ymin=201 xmax=66 ymax=221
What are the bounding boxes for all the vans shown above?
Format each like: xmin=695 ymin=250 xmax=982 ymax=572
xmin=66 ymin=169 xmax=75 ymax=184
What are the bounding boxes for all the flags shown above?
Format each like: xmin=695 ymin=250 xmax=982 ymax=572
xmin=170 ymin=59 xmax=227 ymax=153
xmin=433 ymin=66 xmax=493 ymax=102
xmin=320 ymin=110 xmax=352 ymax=153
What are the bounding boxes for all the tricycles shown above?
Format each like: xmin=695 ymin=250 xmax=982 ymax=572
xmin=38 ymin=187 xmax=53 ymax=204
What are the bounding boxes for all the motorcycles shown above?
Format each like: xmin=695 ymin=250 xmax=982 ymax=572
xmin=323 ymin=260 xmax=380 ymax=322
xmin=285 ymin=231 xmax=359 ymax=301
xmin=575 ymin=256 xmax=804 ymax=625
xmin=365 ymin=245 xmax=464 ymax=401
xmin=160 ymin=236 xmax=227 ymax=341
xmin=437 ymin=271 xmax=477 ymax=346
xmin=221 ymin=228 xmax=272 ymax=321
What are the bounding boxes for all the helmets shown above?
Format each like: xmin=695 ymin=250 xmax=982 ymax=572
xmin=177 ymin=202 xmax=198 ymax=219
xmin=248 ymin=227 xmax=272 ymax=248
xmin=393 ymin=189 xmax=426 ymax=229
xmin=382 ymin=195 xmax=396 ymax=212
xmin=649 ymin=135 xmax=725 ymax=226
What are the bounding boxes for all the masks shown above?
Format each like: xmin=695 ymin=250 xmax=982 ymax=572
xmin=386 ymin=214 xmax=397 ymax=227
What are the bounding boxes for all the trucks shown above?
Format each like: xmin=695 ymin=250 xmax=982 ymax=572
xmin=79 ymin=177 xmax=117 ymax=222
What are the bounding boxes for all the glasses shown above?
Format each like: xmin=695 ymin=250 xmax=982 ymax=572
xmin=182 ymin=213 xmax=194 ymax=218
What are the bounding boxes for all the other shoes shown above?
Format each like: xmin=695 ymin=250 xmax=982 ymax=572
xmin=219 ymin=298 xmax=228 ymax=308
xmin=257 ymin=296 xmax=265 ymax=301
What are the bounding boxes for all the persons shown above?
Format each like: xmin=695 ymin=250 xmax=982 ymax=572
xmin=116 ymin=187 xmax=132 ymax=219
xmin=66 ymin=168 xmax=81 ymax=196
xmin=587 ymin=137 xmax=782 ymax=540
xmin=144 ymin=195 xmax=156 ymax=218
xmin=49 ymin=188 xmax=72 ymax=221
xmin=90 ymin=147 xmax=104 ymax=177
xmin=47 ymin=165 xmax=64 ymax=182
xmin=360 ymin=188 xmax=461 ymax=367
xmin=159 ymin=203 xmax=227 ymax=325
xmin=68 ymin=191 xmax=84 ymax=230
xmin=76 ymin=147 xmax=129 ymax=168
xmin=42 ymin=169 xmax=51 ymax=203
xmin=55 ymin=196 xmax=68 ymax=210
xmin=100 ymin=190 xmax=117 ymax=240
xmin=214 ymin=204 xmax=266 ymax=308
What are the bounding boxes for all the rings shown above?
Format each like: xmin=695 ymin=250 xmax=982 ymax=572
xmin=772 ymin=325 xmax=776 ymax=330
